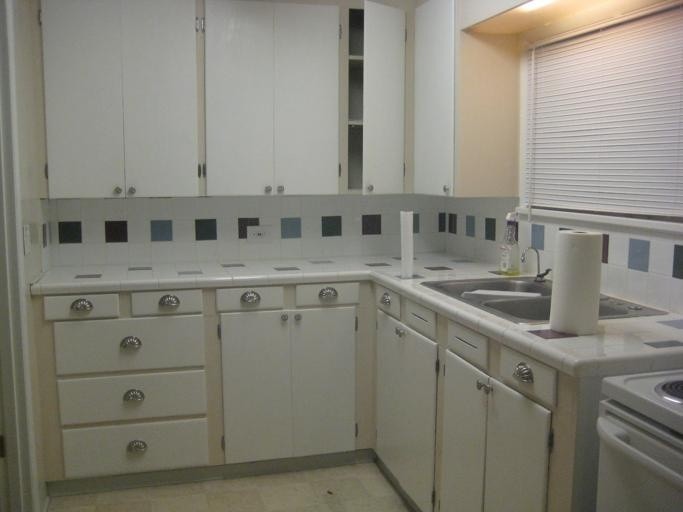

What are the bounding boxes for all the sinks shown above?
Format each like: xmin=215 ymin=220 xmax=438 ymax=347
xmin=434 ymin=274 xmax=553 ymax=302
xmin=484 ymin=295 xmax=632 ymax=323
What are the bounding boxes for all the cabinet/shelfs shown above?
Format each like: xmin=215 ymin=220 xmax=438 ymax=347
xmin=214 ymin=282 xmax=358 ymax=465
xmin=415 ymin=0 xmax=519 ymax=199
xmin=41 ymin=4 xmax=198 ymax=199
xmin=43 ymin=288 xmax=209 ymax=481
xmin=374 ymin=286 xmax=436 ymax=510
xmin=442 ymin=320 xmax=555 ymax=510
xmin=204 ymin=3 xmax=339 ymax=196
xmin=344 ymin=0 xmax=407 ymax=196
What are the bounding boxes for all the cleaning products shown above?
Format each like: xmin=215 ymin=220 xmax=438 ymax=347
xmin=497 ymin=199 xmax=531 ymax=275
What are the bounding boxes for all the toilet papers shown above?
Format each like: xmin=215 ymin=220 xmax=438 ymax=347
xmin=400 ymin=210 xmax=414 ymax=280
xmin=548 ymin=229 xmax=603 ymax=333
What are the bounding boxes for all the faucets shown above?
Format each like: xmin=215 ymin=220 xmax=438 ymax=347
xmin=520 ymin=246 xmax=553 ymax=280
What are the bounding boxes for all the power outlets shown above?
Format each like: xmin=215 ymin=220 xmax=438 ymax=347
xmin=246 ymin=225 xmax=265 ymax=244
